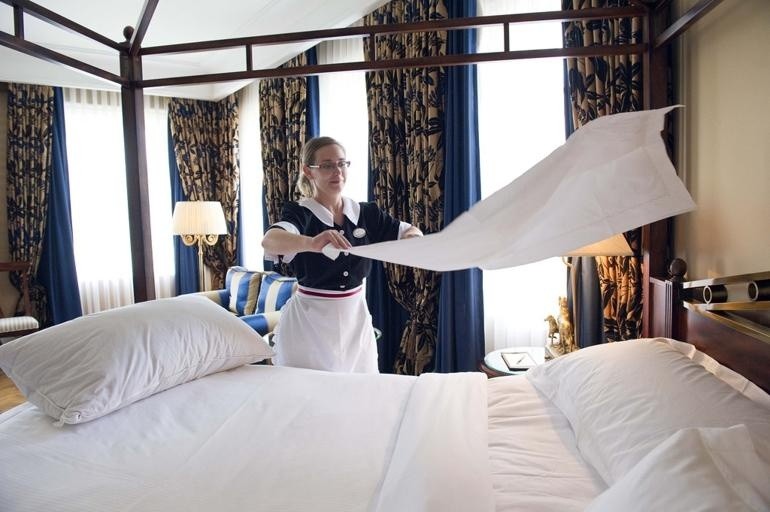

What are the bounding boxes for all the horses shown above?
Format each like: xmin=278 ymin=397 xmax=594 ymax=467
xmin=544 ymin=316 xmax=559 ymax=346
xmin=557 ymin=296 xmax=574 ymax=353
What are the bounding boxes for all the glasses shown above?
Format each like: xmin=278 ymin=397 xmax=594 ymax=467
xmin=308 ymin=161 xmax=351 ymax=169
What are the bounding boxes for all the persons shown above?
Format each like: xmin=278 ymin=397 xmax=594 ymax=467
xmin=261 ymin=135 xmax=425 ymax=376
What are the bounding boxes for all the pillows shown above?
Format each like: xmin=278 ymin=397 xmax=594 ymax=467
xmin=527 ymin=336 xmax=770 ymax=512
xmin=0 ymin=292 xmax=276 ymax=426
xmin=254 ymin=271 xmax=299 ymax=315
xmin=225 ymin=266 xmax=261 ymax=315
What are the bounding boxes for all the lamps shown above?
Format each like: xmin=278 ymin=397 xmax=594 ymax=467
xmin=563 ymin=233 xmax=634 ymax=256
xmin=169 ymin=200 xmax=230 ymax=294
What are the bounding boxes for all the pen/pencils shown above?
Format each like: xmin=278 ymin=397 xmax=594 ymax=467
xmin=516 ymin=355 xmax=527 ymax=364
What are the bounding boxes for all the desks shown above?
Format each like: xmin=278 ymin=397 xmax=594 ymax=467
xmin=545 ymin=342 xmax=581 ymax=358
xmin=479 ymin=347 xmax=557 ymax=376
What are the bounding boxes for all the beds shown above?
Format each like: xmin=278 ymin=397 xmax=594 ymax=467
xmin=0 ymin=257 xmax=769 ymax=512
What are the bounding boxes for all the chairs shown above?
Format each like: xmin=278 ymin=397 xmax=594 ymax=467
xmin=176 ymin=265 xmax=300 ymax=332
xmin=0 ymin=261 xmax=38 ymax=345
